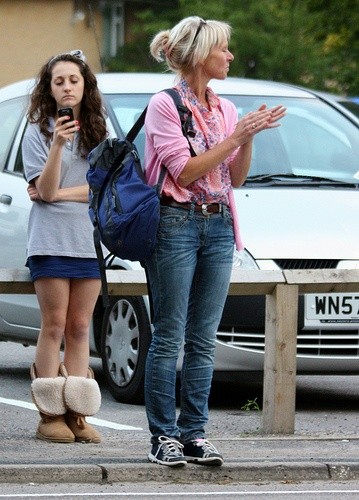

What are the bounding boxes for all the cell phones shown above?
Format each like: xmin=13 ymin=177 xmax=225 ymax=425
xmin=58 ymin=108 xmax=75 ymax=133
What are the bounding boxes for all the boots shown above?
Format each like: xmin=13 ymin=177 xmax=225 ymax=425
xmin=30 ymin=362 xmax=75 ymax=443
xmin=60 ymin=363 xmax=102 ymax=442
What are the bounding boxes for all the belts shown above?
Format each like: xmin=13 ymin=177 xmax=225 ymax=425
xmin=160 ymin=195 xmax=224 ymax=216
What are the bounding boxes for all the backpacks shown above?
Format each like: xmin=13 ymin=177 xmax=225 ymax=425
xmin=84 ymin=88 xmax=196 ymax=307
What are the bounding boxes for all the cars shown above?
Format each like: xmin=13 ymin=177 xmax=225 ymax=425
xmin=0 ymin=71 xmax=359 ymax=405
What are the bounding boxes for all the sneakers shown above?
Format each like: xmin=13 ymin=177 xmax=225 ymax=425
xmin=148 ymin=435 xmax=188 ymax=465
xmin=182 ymin=438 xmax=223 ymax=467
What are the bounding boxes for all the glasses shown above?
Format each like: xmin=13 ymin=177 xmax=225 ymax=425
xmin=192 ymin=18 xmax=206 ymax=45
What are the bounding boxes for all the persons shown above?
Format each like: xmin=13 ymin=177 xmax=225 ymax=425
xmin=20 ymin=50 xmax=106 ymax=443
xmin=143 ymin=16 xmax=286 ymax=468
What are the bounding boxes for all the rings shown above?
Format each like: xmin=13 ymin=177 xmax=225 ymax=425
xmin=252 ymin=123 xmax=256 ymax=129
